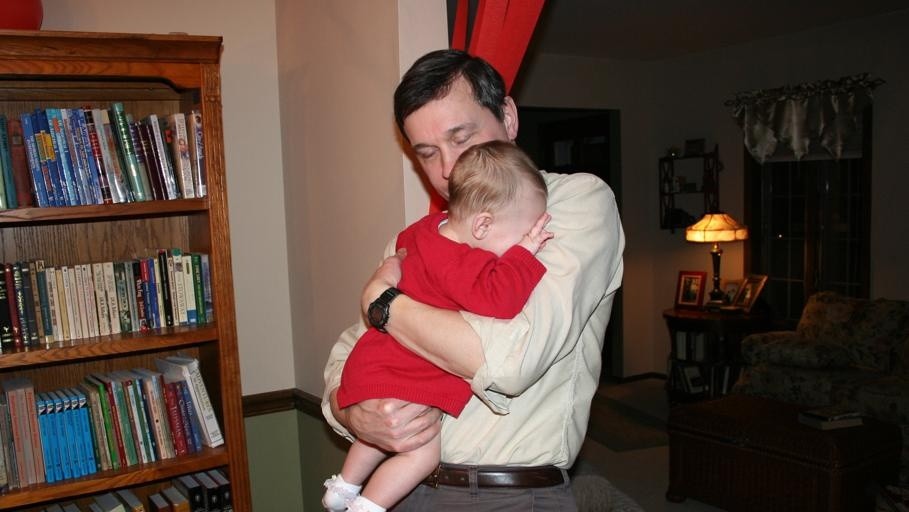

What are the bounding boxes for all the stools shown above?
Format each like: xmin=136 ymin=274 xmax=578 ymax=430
xmin=667 ymin=394 xmax=891 ymax=509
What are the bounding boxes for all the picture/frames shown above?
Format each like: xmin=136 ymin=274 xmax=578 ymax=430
xmin=734 ymin=276 xmax=767 ymax=312
xmin=676 ymin=273 xmax=707 ymax=306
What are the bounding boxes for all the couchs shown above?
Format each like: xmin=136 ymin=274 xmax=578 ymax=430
xmin=737 ymin=291 xmax=905 ymax=418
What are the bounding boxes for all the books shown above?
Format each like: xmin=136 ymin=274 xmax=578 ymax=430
xmin=0 ymin=248 xmax=212 ymax=349
xmin=33 ymin=471 xmax=233 ymax=512
xmin=676 ymin=367 xmax=711 ymax=395
xmin=0 ymin=80 xmax=208 ymax=208
xmin=0 ymin=355 xmax=220 ymax=489
xmin=675 ymin=332 xmax=710 ymax=363
xmin=795 ymin=401 xmax=865 ymax=433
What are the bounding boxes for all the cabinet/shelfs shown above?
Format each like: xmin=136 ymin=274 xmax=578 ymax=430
xmin=0 ymin=31 xmax=251 ymax=509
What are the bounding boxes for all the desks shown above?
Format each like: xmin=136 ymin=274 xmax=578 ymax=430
xmin=662 ymin=309 xmax=768 ymax=395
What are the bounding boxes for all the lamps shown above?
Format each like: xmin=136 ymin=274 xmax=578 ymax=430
xmin=685 ymin=215 xmax=741 ymax=307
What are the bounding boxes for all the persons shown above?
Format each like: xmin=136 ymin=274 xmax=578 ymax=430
xmin=322 ymin=140 xmax=553 ymax=512
xmin=321 ymin=49 xmax=622 ymax=510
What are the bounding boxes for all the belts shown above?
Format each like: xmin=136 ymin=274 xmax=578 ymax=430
xmin=421 ymin=463 xmax=567 ymax=489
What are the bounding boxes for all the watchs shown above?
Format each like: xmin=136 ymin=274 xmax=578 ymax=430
xmin=365 ymin=287 xmax=400 ymax=331
